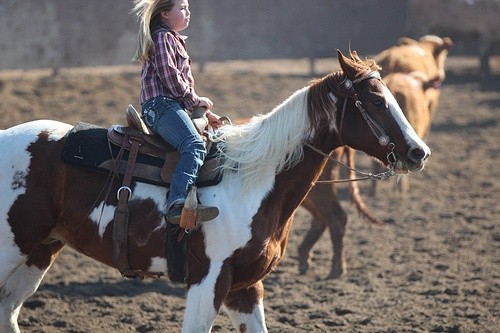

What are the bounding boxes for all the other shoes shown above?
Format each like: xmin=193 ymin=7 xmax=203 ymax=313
xmin=165 ymin=202 xmax=219 ymax=224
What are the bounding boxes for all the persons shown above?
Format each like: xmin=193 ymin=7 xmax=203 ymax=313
xmin=128 ymin=0 xmax=225 ymax=225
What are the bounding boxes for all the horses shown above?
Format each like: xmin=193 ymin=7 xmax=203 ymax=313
xmin=373 ymin=35 xmax=453 ymax=123
xmin=230 ymin=117 xmax=384 ymax=279
xmin=0 ymin=48 xmax=432 ymax=333
xmin=329 ymin=71 xmax=442 ymax=224
